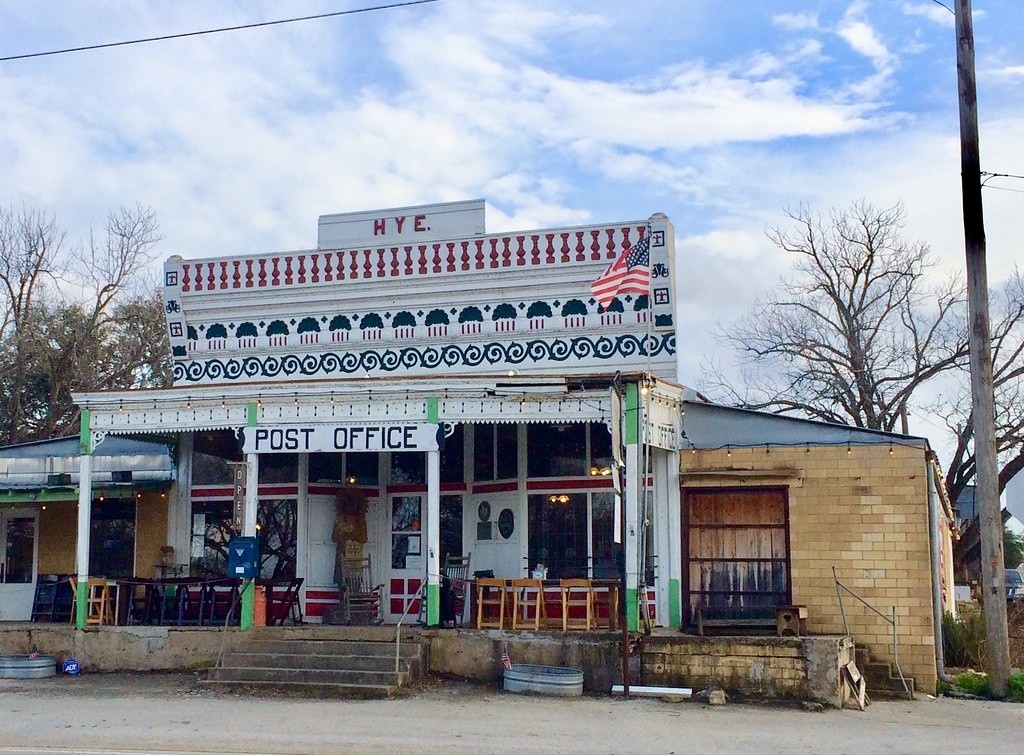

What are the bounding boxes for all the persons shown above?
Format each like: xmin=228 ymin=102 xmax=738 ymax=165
xmin=332 ymin=509 xmax=368 ymax=601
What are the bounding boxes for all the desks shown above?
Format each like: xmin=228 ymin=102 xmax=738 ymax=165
xmin=118 ymin=578 xmax=277 ymax=626
xmin=90 ymin=580 xmax=133 ymax=625
xmin=461 ymin=578 xmax=621 ymax=632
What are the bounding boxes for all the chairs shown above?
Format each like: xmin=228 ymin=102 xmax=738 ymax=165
xmin=268 ymin=577 xmax=305 ymax=626
xmin=128 ymin=584 xmax=237 ymax=627
xmin=68 ymin=577 xmax=114 ymax=627
xmin=559 ymin=578 xmax=598 ymax=631
xmin=475 ymin=577 xmax=512 ymax=630
xmin=417 ymin=552 xmax=472 ymax=628
xmin=510 ymin=578 xmax=548 ymax=632
xmin=31 ymin=573 xmax=77 ymax=623
xmin=339 ymin=553 xmax=386 ymax=625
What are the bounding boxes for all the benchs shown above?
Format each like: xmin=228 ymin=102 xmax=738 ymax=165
xmin=776 ymin=607 xmax=808 ymax=635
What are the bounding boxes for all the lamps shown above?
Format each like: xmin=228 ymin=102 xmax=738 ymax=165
xmin=558 ymin=495 xmax=569 ymax=503
xmin=548 ymin=496 xmax=558 ymax=502
xmin=590 ymin=467 xmax=612 ymax=476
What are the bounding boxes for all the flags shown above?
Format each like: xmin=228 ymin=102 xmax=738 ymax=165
xmin=591 ymin=237 xmax=650 ymax=312
